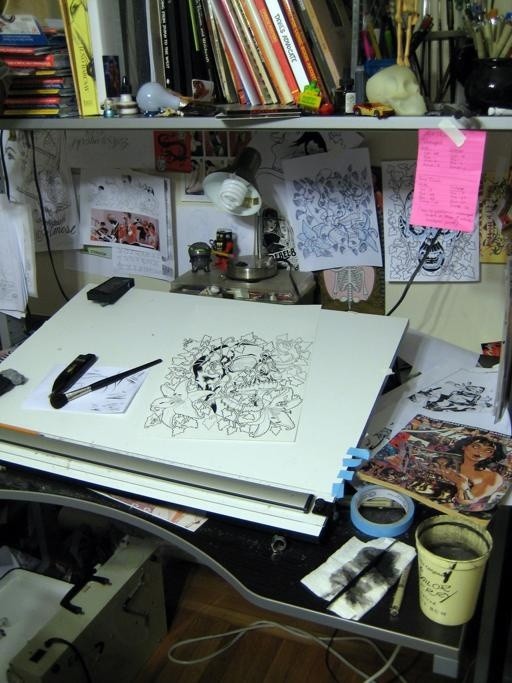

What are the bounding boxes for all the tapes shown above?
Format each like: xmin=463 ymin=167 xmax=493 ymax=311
xmin=350 ymin=485 xmax=415 ymax=538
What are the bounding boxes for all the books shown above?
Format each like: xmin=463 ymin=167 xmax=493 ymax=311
xmin=0 ymin=33 xmax=79 ymax=119
xmin=145 ymin=1 xmax=353 ymax=106
xmin=353 ymin=412 xmax=511 ymax=529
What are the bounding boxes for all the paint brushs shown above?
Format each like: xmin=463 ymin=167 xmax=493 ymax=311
xmin=461 ymin=5 xmax=512 ymax=61
xmin=51 ymin=359 xmax=162 ymax=410
xmin=323 ymin=533 xmax=410 ymax=609
xmin=360 ymin=0 xmax=434 ymax=60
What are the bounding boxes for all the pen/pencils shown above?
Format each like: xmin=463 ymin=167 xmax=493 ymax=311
xmin=390 ymin=561 xmax=413 ymax=617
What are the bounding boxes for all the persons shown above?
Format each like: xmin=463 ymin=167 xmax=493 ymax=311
xmin=259 ymin=206 xmax=289 ymax=252
xmin=89 ymin=210 xmax=162 ymax=252
xmin=360 ymin=415 xmax=504 ymax=513
xmin=157 ymin=131 xmax=229 ymax=195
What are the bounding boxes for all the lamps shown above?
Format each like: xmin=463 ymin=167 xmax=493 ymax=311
xmin=202 ymin=147 xmax=278 ymax=283
xmin=136 ymin=82 xmax=221 ymax=117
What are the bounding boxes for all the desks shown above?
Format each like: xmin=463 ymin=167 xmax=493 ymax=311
xmin=0 ymin=313 xmax=512 ymax=682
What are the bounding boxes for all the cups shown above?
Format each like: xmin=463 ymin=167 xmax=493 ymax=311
xmin=415 ymin=515 xmax=494 ymax=627
